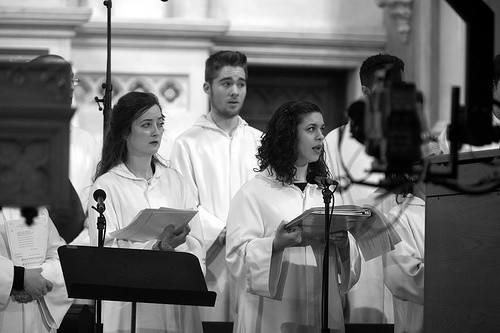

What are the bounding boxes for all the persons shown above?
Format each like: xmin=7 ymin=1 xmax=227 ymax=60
xmin=324 ymin=52 xmax=500 ymax=333
xmin=170 ymin=51 xmax=266 ymax=321
xmin=224 ymin=100 xmax=361 ymax=333
xmin=0 ymin=206 xmax=74 ymax=333
xmin=87 ymin=92 xmax=207 ymax=333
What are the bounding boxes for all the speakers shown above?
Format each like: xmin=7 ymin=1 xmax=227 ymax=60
xmin=1 ymin=60 xmax=73 ymax=209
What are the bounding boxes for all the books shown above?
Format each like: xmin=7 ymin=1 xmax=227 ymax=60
xmin=285 ymin=204 xmax=403 ymax=261
xmin=107 ymin=206 xmax=199 ymax=243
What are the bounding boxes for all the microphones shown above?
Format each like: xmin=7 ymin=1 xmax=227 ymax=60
xmin=93 ymin=189 xmax=107 ymax=212
xmin=306 ymin=172 xmax=338 ymax=186
